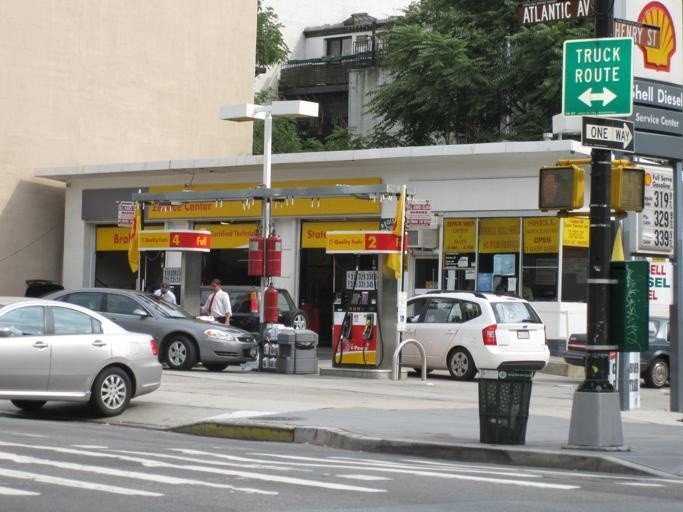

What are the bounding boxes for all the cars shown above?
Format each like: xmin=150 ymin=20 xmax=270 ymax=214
xmin=401 ymin=289 xmax=551 ymax=381
xmin=0 ymin=295 xmax=162 ymax=415
xmin=41 ymin=287 xmax=258 ymax=371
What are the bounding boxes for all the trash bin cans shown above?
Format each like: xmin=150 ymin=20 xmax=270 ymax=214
xmin=475 ymin=374 xmax=534 ymax=446
xmin=276 ymin=328 xmax=319 ymax=375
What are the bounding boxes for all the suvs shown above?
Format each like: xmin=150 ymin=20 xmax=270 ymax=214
xmin=200 ymin=285 xmax=308 ymax=348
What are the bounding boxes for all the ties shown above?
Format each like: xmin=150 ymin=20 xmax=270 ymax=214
xmin=208 ymin=292 xmax=217 ymax=314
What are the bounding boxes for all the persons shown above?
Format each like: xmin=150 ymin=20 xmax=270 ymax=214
xmin=517 ymin=280 xmax=535 ymax=300
xmin=199 ymin=278 xmax=233 ymax=326
xmin=153 ymin=282 xmax=177 ymax=305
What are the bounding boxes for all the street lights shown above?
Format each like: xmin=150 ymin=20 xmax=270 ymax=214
xmin=218 ymin=100 xmax=319 ymax=373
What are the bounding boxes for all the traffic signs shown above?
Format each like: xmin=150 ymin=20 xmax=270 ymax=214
xmin=518 ymin=2 xmax=610 ymax=24
xmin=612 ymin=17 xmax=661 ymax=49
xmin=564 ymin=40 xmax=629 ymax=117
xmin=562 ymin=37 xmax=634 ymax=118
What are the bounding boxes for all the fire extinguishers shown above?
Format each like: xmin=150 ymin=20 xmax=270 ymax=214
xmin=264 ymin=282 xmax=278 ymax=323
xmin=266 ymin=222 xmax=282 ymax=277
xmin=248 ymin=221 xmax=263 ymax=276
